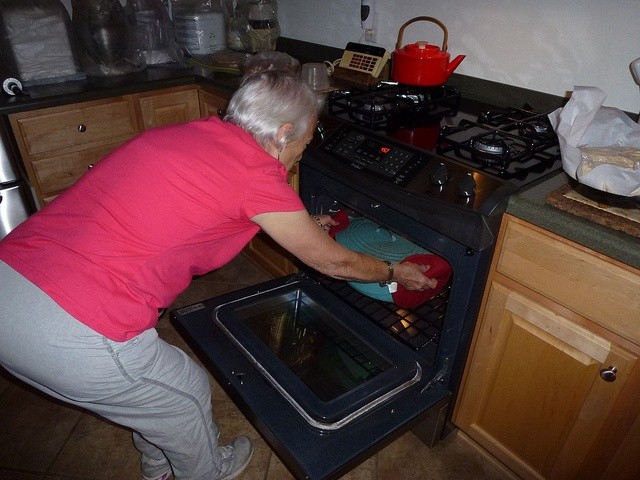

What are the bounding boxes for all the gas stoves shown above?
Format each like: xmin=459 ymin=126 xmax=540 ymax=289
xmin=298 ymin=83 xmax=571 ymax=252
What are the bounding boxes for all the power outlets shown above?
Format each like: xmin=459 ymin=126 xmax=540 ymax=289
xmin=364 ymin=13 xmax=378 ymax=43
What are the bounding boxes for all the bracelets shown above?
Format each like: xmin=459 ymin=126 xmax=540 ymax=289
xmin=379 ymin=260 xmax=394 ymax=287
xmin=309 ymin=214 xmax=323 ymax=229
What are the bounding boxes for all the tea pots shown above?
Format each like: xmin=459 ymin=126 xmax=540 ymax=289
xmin=385 ymin=14 xmax=468 ymax=86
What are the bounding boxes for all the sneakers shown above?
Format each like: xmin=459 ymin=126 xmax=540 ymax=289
xmin=216 ymin=435 xmax=254 ymax=480
xmin=139 ymin=422 xmax=221 ymax=480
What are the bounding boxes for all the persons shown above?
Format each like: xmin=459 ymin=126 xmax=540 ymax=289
xmin=1 ymin=66 xmax=439 ymax=480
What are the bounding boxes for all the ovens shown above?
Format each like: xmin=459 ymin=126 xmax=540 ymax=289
xmin=169 ymin=160 xmax=481 ymax=476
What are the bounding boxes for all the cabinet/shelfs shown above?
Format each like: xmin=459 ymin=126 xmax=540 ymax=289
xmin=197 ymin=90 xmax=232 ymax=126
xmin=449 ymin=212 xmax=640 ymax=480
xmin=139 ymin=86 xmax=203 ymax=132
xmin=7 ymin=93 xmax=139 ymax=211
